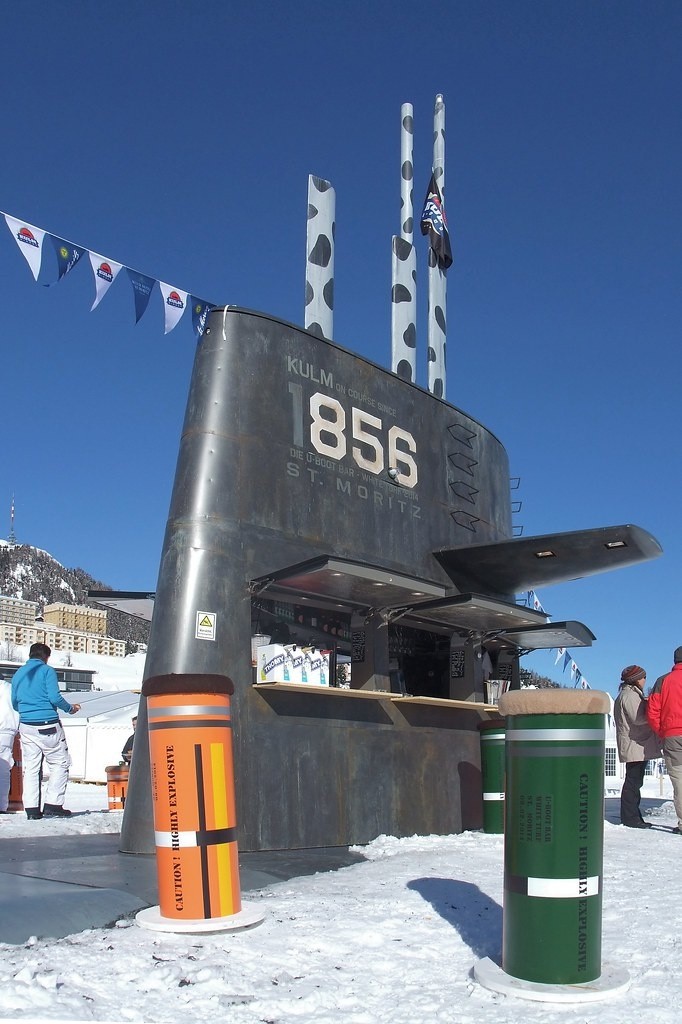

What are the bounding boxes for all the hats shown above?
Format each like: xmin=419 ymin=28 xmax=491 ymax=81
xmin=674 ymin=646 xmax=682 ymax=662
xmin=621 ymin=665 xmax=646 ymax=684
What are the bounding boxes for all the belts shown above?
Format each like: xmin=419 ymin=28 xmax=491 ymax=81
xmin=21 ymin=719 xmax=59 ymax=726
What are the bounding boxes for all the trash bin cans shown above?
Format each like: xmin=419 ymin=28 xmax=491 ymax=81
xmin=105 ymin=765 xmax=130 ymax=810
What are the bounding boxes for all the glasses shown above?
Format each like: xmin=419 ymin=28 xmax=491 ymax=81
xmin=644 ymin=676 xmax=646 ymax=679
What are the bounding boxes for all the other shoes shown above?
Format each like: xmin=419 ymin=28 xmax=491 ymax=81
xmin=0 ymin=809 xmax=16 ymax=814
xmin=672 ymin=827 xmax=682 ymax=835
xmin=636 ymin=821 xmax=652 ymax=828
xmin=25 ymin=807 xmax=42 ymax=820
xmin=43 ymin=803 xmax=74 ymax=819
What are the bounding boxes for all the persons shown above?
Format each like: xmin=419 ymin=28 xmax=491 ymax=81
xmin=11 ymin=643 xmax=78 ymax=820
xmin=645 ymin=646 xmax=682 ymax=835
xmin=121 ymin=716 xmax=138 ymax=765
xmin=0 ymin=673 xmax=20 ymax=814
xmin=613 ymin=665 xmax=663 ymax=829
